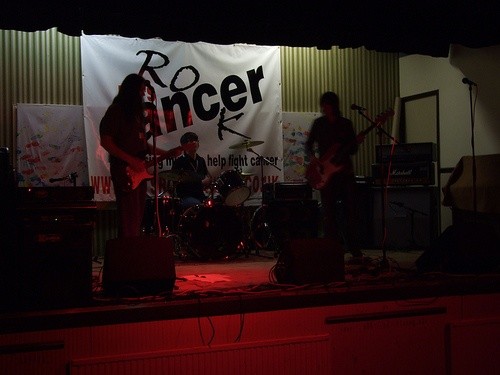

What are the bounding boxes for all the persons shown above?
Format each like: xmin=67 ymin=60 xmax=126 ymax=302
xmin=0 ymin=148 xmax=39 ymax=281
xmin=304 ymin=91 xmax=365 ymax=255
xmin=101 ymin=74 xmax=180 ymax=235
xmin=171 ymin=133 xmax=212 ymax=207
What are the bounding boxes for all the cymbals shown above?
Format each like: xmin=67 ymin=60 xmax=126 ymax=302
xmin=240 ymin=172 xmax=255 ymax=176
xmin=229 ymin=141 xmax=265 ymax=150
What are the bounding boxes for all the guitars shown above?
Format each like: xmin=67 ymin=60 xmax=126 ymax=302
xmin=305 ymin=106 xmax=395 ymax=190
xmin=121 ymin=141 xmax=197 ymax=200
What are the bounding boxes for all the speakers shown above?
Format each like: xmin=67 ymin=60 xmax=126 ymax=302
xmin=266 ymin=180 xmax=500 ymax=285
xmin=103 ymin=237 xmax=176 ymax=297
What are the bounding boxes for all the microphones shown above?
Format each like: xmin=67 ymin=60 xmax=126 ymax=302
xmin=49 ymin=178 xmax=64 ymax=182
xmin=350 ymin=104 xmax=366 ymax=110
xmin=462 ymin=78 xmax=477 ymax=87
xmin=143 ymin=80 xmax=154 ymax=91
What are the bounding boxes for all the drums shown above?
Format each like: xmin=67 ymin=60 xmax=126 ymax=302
xmin=147 ymin=195 xmax=183 ymax=237
xmin=216 ymin=168 xmax=251 ymax=207
xmin=199 ymin=187 xmax=225 ymax=208
xmin=179 ymin=205 xmax=225 ymax=261
